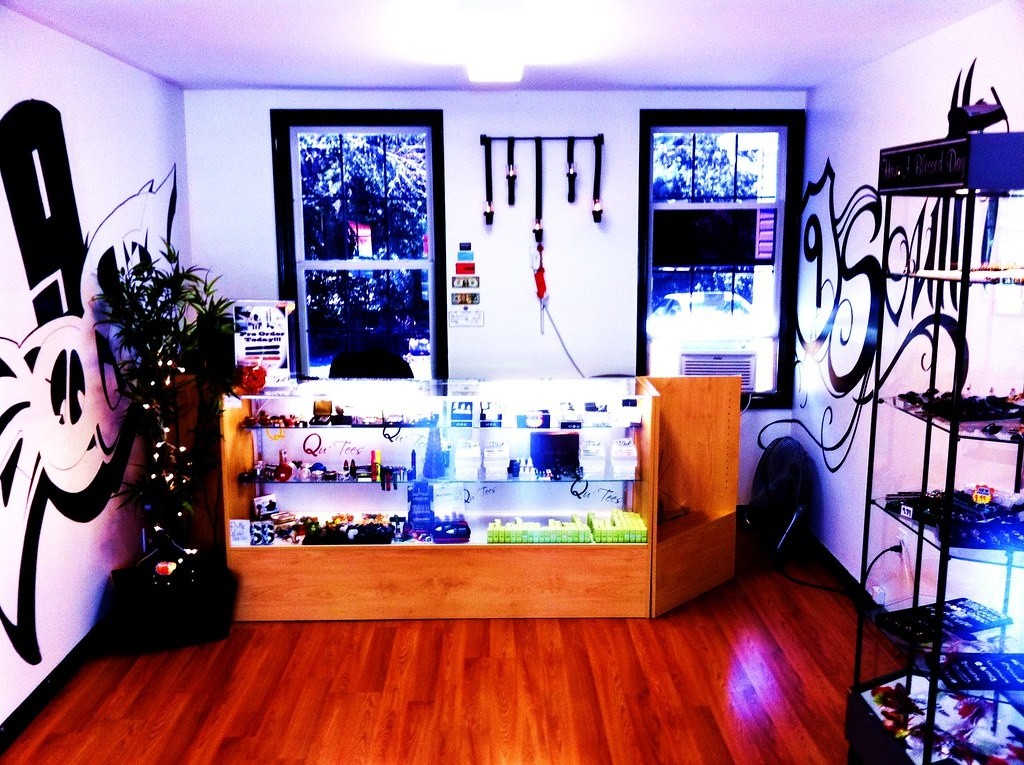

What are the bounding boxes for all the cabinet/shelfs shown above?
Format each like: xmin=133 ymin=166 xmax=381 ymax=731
xmin=843 ymin=129 xmax=1024 ymax=765
xmin=211 ymin=373 xmax=740 ymax=621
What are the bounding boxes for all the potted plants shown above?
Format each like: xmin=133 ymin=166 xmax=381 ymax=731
xmin=96 ymin=240 xmax=257 ymax=653
